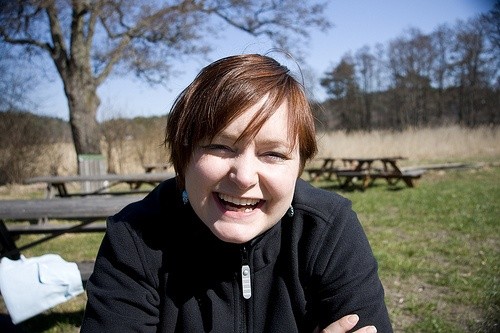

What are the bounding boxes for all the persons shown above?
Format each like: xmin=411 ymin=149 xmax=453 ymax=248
xmin=80 ymin=54 xmax=393 ymax=333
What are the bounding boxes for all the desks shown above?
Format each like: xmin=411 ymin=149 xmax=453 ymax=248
xmin=0 ymin=199 xmax=149 ymax=261
xmin=342 ymin=156 xmax=403 ymax=189
xmin=319 ymin=157 xmax=335 ymax=183
xmin=31 ymin=175 xmax=177 ymax=199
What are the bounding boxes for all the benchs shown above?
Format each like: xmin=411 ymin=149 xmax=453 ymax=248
xmin=8 ymin=225 xmax=109 ymax=241
xmin=144 ymin=164 xmax=171 ymax=174
xmin=306 ymin=168 xmax=322 ymax=174
xmin=335 ymin=168 xmax=371 ymax=178
xmin=0 ymin=262 xmax=94 ymax=296
xmin=385 ymin=171 xmax=421 ymax=180
